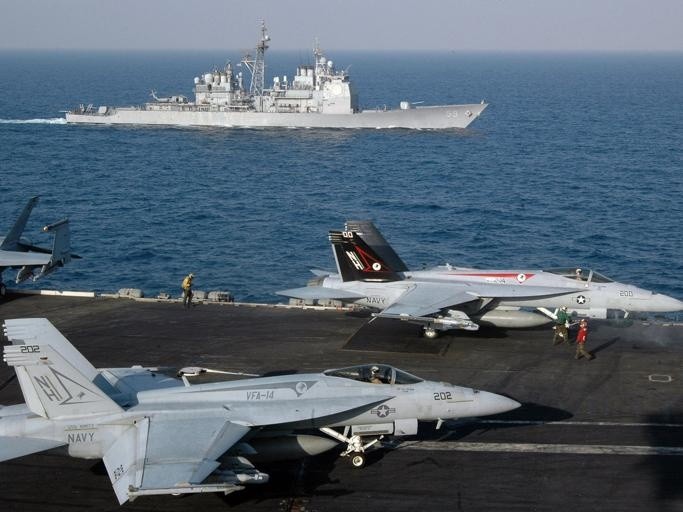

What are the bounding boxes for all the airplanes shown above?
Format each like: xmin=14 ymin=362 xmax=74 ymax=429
xmin=273 ymin=219 xmax=683 ymax=339
xmin=0 ymin=195 xmax=84 ymax=297
xmin=0 ymin=318 xmax=521 ymax=506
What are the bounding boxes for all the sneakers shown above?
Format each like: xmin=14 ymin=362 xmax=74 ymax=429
xmin=574 ymin=355 xmax=593 ymax=361
xmin=552 ymin=340 xmax=571 ymax=346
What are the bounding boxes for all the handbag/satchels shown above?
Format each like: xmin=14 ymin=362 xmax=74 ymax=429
xmin=185 ymin=286 xmax=192 ymax=298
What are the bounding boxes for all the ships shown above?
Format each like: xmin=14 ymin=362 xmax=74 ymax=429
xmin=64 ymin=17 xmax=489 ymax=129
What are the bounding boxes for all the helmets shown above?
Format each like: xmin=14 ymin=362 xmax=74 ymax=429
xmin=562 ymin=306 xmax=568 ymax=311
xmin=580 ymin=318 xmax=588 ymax=327
xmin=189 ymin=273 xmax=195 ymax=279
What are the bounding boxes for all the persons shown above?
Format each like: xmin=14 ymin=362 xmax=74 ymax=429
xmin=551 ymin=307 xmax=575 ymax=345
xmin=368 ymin=366 xmax=386 ymax=384
xmin=573 ymin=317 xmax=593 ymax=359
xmin=182 ymin=272 xmax=196 ymax=309
xmin=575 ymin=268 xmax=583 ymax=281
